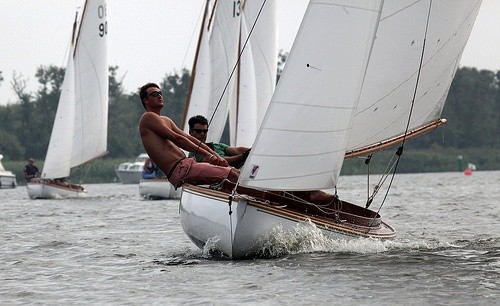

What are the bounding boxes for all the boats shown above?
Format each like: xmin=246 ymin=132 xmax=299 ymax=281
xmin=0 ymin=152 xmax=19 ymax=189
xmin=115 ymin=153 xmax=160 ymax=184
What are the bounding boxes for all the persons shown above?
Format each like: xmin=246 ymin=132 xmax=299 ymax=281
xmin=188 ymin=115 xmax=335 ymax=201
xmin=139 ymin=83 xmax=242 ymax=188
xmin=24 ymin=158 xmax=41 ymax=183
xmin=143 ymin=158 xmax=167 ymax=179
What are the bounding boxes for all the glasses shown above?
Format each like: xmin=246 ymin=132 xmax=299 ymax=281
xmin=146 ymin=90 xmax=163 ymax=99
xmin=191 ymin=128 xmax=208 ymax=133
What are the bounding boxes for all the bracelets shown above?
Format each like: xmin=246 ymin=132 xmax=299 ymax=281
xmin=208 ymin=155 xmax=214 ymax=160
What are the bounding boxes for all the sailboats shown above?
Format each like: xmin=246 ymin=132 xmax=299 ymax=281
xmin=139 ymin=0 xmax=281 ymax=200
xmin=25 ymin=0 xmax=111 ymax=200
xmin=177 ymin=0 xmax=485 ymax=259
